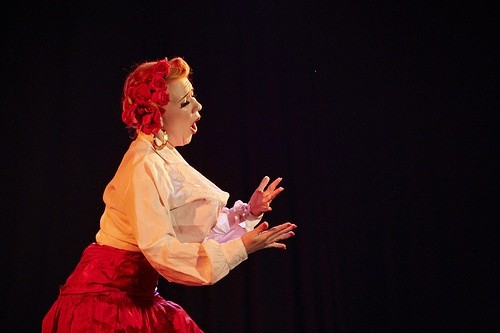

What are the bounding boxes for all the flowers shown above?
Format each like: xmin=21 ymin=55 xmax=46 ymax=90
xmin=121 ymin=59 xmax=172 ymax=136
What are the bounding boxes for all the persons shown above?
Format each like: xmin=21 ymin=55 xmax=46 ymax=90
xmin=42 ymin=57 xmax=298 ymax=333
